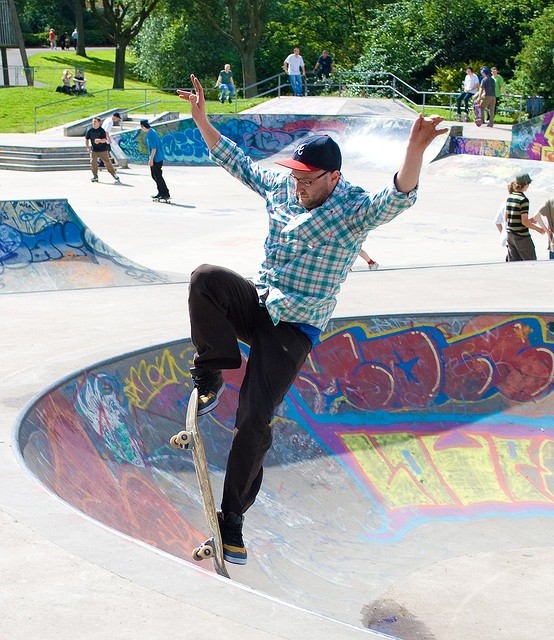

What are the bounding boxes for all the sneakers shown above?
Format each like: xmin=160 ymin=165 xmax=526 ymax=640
xmin=198 ymin=373 xmax=226 ymax=416
xmin=368 ymin=260 xmax=379 ymax=273
xmin=159 ymin=192 xmax=170 ymax=199
xmin=152 ymin=193 xmax=162 ymax=198
xmin=218 ymin=513 xmax=249 ymax=567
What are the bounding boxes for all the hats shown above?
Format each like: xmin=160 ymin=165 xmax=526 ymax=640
xmin=112 ymin=112 xmax=122 ymax=120
xmin=140 ymin=118 xmax=152 ymax=129
xmin=516 ymin=172 xmax=533 ymax=185
xmin=274 ymin=133 xmax=343 ymax=173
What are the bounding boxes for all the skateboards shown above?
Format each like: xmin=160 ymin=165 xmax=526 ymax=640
xmin=473 ymin=99 xmax=482 ymax=127
xmin=169 ymin=388 xmax=230 ymax=580
xmin=151 ymin=196 xmax=173 ymax=203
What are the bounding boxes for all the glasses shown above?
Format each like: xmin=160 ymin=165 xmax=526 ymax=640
xmin=289 ymin=172 xmax=328 ymax=185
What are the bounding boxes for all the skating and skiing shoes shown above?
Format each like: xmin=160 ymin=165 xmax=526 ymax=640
xmin=113 ymin=175 xmax=120 ymax=182
xmin=91 ymin=176 xmax=99 ymax=183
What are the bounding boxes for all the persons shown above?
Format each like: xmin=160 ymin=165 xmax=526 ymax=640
xmin=140 ymin=119 xmax=170 ymax=199
xmin=347 ymin=248 xmax=379 ymax=272
xmin=59 ymin=31 xmax=69 ymax=50
xmin=496 ymin=183 xmax=515 ymax=263
xmin=472 ymin=65 xmax=489 ymax=118
xmin=283 ymin=47 xmax=305 ymax=96
xmin=72 ymin=29 xmax=78 ymax=49
xmin=97 ymin=112 xmax=122 ymax=167
xmin=176 ymin=73 xmax=448 ymax=566
xmin=85 ymin=117 xmax=119 ymax=181
xmin=486 ymin=66 xmax=507 ymax=120
xmin=72 ymin=70 xmax=87 ymax=92
xmin=505 ymin=174 xmax=548 ymax=262
xmin=452 ymin=67 xmax=480 ymax=118
xmin=311 ymin=50 xmax=334 ymax=88
xmin=215 ymin=64 xmax=235 ymax=104
xmin=476 ymin=67 xmax=496 ymax=127
xmin=49 ymin=29 xmax=57 ymax=50
xmin=536 ymin=196 xmax=554 ymax=260
xmin=61 ymin=69 xmax=76 ymax=92
